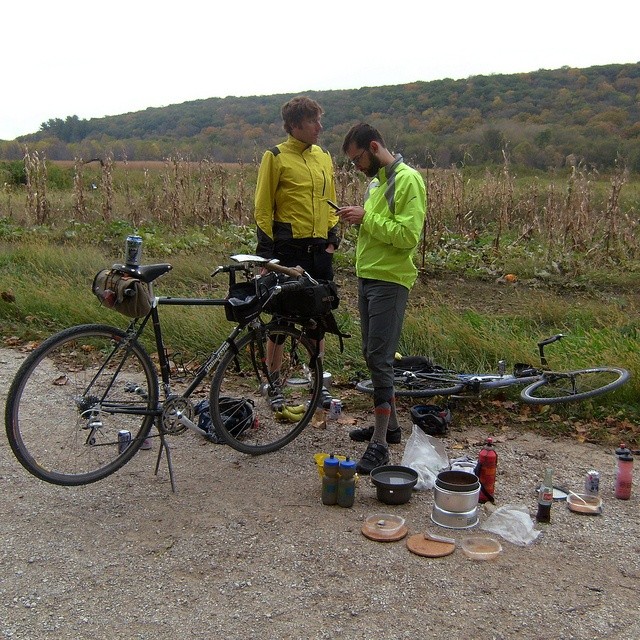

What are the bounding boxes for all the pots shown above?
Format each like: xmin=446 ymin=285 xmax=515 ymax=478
xmin=369 ymin=463 xmax=420 ymax=505
xmin=430 ymin=468 xmax=482 ymax=528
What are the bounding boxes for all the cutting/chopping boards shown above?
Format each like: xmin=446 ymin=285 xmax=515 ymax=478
xmin=360 ymin=519 xmax=409 ymax=544
xmin=405 ymin=532 xmax=455 ymax=559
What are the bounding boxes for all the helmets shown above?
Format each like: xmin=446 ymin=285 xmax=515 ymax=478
xmin=410 ymin=404 xmax=450 ymax=436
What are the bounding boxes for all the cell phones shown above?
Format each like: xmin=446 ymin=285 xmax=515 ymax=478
xmin=327 ymin=200 xmax=341 ymax=211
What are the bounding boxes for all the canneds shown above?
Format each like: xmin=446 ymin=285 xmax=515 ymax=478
xmin=585 ymin=470 xmax=599 ymax=496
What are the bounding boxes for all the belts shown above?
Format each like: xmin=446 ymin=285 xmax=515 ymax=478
xmin=307 ymin=245 xmax=321 ymax=253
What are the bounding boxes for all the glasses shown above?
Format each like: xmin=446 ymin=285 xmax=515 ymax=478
xmin=353 ymin=149 xmax=366 ymax=165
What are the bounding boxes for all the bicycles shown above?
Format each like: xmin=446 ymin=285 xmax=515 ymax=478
xmin=5 ymin=255 xmax=324 ymax=492
xmin=357 ymin=334 xmax=629 ymax=404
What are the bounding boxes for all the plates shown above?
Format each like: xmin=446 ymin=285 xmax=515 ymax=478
xmin=565 ymin=492 xmax=604 ymax=517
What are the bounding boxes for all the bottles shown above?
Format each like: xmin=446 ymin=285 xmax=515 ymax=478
xmin=321 ymin=453 xmax=338 ymax=504
xmin=615 ymin=443 xmax=631 ymax=464
xmin=613 ymin=449 xmax=634 ymax=501
xmin=337 ymin=456 xmax=356 ymax=509
xmin=477 ymin=441 xmax=499 ymax=505
xmin=536 ymin=465 xmax=556 ymax=524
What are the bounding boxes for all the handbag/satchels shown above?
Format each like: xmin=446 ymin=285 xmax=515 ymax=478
xmin=251 ymin=272 xmax=351 ymax=354
xmin=195 ymin=398 xmax=254 ymax=444
xmin=92 ymin=269 xmax=152 ymax=318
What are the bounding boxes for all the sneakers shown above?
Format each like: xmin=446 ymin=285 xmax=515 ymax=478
xmin=312 ymin=384 xmax=334 ymax=409
xmin=355 ymin=441 xmax=393 ymax=475
xmin=268 ymin=384 xmax=286 ymax=412
xmin=348 ymin=426 xmax=402 ymax=444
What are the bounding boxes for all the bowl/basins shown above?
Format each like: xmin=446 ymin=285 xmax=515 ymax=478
xmin=459 ymin=535 xmax=504 ymax=561
xmin=365 ymin=512 xmax=406 ymax=537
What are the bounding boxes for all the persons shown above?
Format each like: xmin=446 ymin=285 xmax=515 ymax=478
xmin=335 ymin=121 xmax=427 ymax=472
xmin=253 ymin=96 xmax=339 ymax=409
